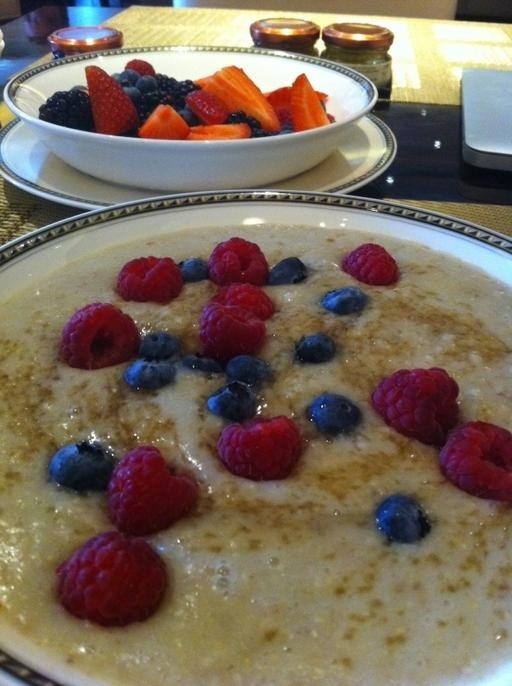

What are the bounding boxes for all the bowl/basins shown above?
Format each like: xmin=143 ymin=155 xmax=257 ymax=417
xmin=1 ymin=190 xmax=512 ymax=686
xmin=3 ymin=46 xmax=378 ymax=197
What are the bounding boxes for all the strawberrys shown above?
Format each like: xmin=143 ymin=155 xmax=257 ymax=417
xmin=85 ymin=59 xmax=337 ymax=140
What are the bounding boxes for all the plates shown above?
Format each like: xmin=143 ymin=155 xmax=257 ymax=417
xmin=2 ymin=114 xmax=398 ymax=212
